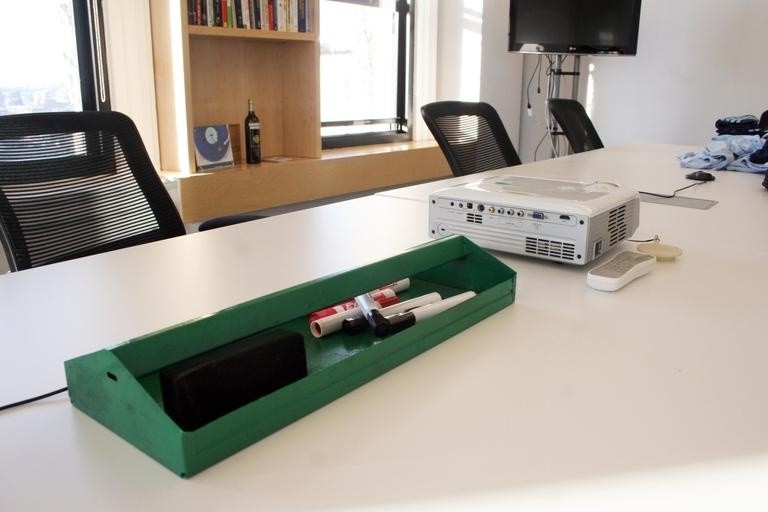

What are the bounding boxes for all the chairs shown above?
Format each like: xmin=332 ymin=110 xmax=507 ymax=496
xmin=0 ymin=112 xmax=186 ymax=275
xmin=420 ymin=102 xmax=520 ymax=176
xmin=547 ymin=98 xmax=604 ymax=152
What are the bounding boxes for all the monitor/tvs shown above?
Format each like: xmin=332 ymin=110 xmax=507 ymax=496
xmin=508 ymin=0 xmax=641 ymax=55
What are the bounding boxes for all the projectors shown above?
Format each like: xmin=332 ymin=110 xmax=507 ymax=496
xmin=426 ymin=172 xmax=642 ymax=267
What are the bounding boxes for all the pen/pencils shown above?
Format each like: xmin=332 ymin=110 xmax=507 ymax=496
xmin=306 ymin=277 xmax=476 ymax=339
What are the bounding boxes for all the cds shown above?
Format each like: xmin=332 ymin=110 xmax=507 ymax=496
xmin=195 ymin=125 xmax=229 ymax=162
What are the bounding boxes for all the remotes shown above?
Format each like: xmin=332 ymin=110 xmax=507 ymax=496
xmin=586 ymin=251 xmax=656 ymax=294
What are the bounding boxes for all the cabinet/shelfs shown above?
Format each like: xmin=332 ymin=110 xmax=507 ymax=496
xmin=149 ymin=1 xmax=321 ymax=172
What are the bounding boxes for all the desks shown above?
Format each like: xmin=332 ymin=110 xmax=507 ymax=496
xmin=0 ymin=142 xmax=768 ymax=512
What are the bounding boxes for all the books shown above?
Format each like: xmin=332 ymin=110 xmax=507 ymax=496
xmin=189 ymin=0 xmax=311 ymax=33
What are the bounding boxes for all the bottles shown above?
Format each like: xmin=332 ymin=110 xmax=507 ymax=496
xmin=245 ymin=98 xmax=261 ymax=164
